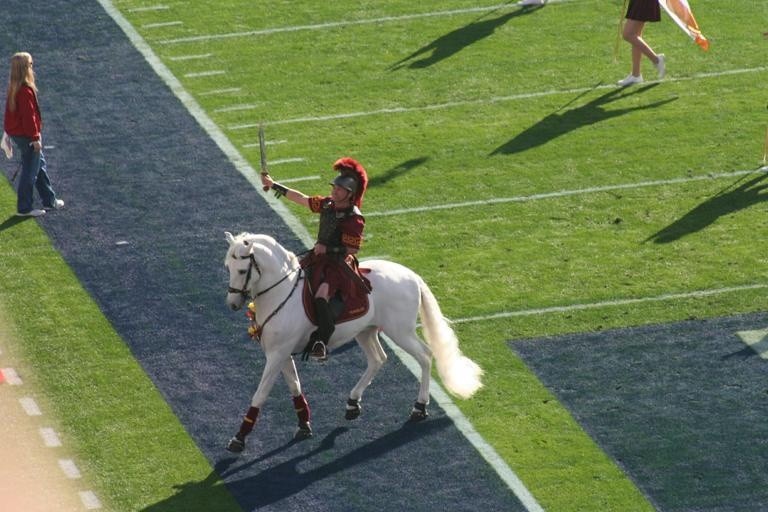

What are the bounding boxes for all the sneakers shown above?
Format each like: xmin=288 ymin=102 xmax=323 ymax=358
xmin=17 ymin=209 xmax=46 ymax=218
xmin=618 ymin=72 xmax=643 ymax=85
xmin=44 ymin=197 xmax=64 ymax=210
xmin=655 ymin=53 xmax=665 ymax=79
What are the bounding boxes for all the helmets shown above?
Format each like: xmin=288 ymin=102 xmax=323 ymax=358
xmin=329 ymin=158 xmax=367 ymax=208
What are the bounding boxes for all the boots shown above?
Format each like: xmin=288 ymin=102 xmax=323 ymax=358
xmin=309 ymin=297 xmax=335 ymax=350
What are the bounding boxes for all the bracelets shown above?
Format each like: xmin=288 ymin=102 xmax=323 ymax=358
xmin=326 ymin=245 xmax=347 ymax=255
xmin=272 ymin=183 xmax=289 ymax=197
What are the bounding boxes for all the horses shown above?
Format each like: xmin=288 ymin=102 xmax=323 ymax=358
xmin=223 ymin=231 xmax=485 ymax=454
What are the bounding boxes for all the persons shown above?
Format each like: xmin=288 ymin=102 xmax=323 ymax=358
xmin=617 ymin=0 xmax=665 ymax=86
xmin=261 ymin=158 xmax=368 ymax=349
xmin=4 ymin=52 xmax=64 ymax=216
